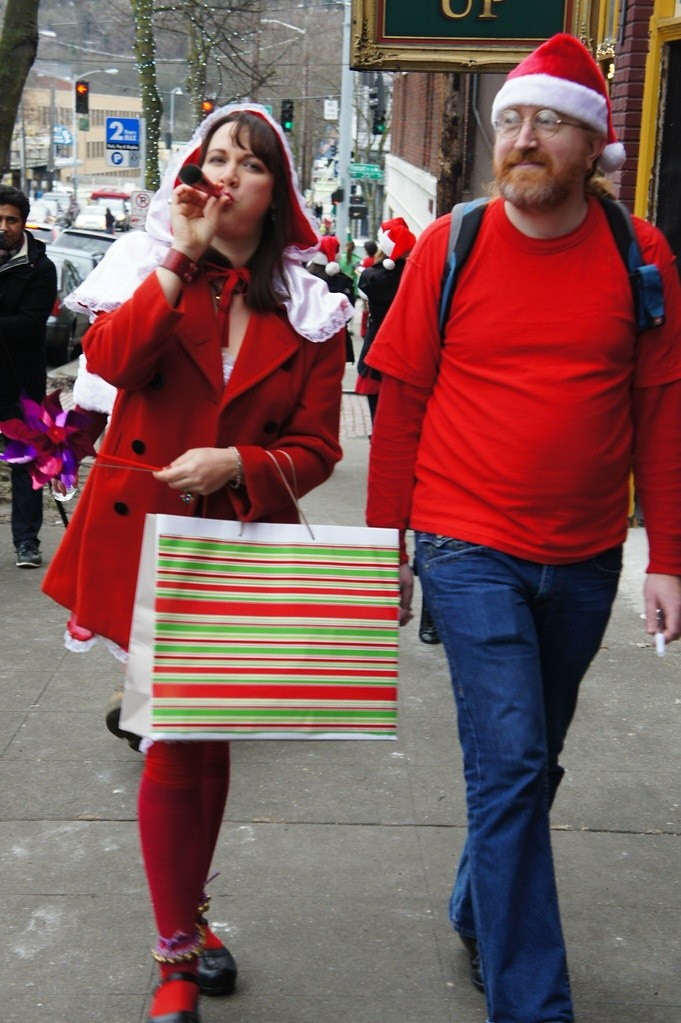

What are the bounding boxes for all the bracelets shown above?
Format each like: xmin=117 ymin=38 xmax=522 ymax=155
xmin=160 ymin=247 xmax=199 ymax=284
xmin=230 ymin=447 xmax=241 ymax=489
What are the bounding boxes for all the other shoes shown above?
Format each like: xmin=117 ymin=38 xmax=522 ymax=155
xmin=105 ymin=709 xmax=143 ymax=755
xmin=461 ymin=932 xmax=486 ymax=992
xmin=146 ymin=977 xmax=199 ymax=1023
xmin=193 ymin=935 xmax=236 ymax=994
xmin=418 ymin=586 xmax=441 ymax=642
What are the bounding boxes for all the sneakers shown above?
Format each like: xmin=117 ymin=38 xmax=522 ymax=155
xmin=15 ymin=539 xmax=41 ymax=569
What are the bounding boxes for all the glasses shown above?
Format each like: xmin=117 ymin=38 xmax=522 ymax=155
xmin=494 ymin=109 xmax=592 ymax=139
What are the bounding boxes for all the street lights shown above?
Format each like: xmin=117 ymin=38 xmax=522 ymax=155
xmin=18 ymin=29 xmax=57 ymax=197
xmin=169 ymin=86 xmax=184 ymax=132
xmin=36 ymin=72 xmax=56 ymax=193
xmin=259 ymin=18 xmax=309 ymax=197
xmin=72 ymin=68 xmax=120 ymax=191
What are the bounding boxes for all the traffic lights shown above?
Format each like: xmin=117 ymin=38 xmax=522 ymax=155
xmin=371 ymin=110 xmax=387 ymax=135
xmin=331 ymin=189 xmax=343 ymax=205
xmin=280 ymin=100 xmax=294 ymax=134
xmin=350 ymin=195 xmax=364 ymax=206
xmin=75 ymin=80 xmax=89 ymax=114
xmin=349 ymin=206 xmax=368 ymax=220
xmin=351 ymin=185 xmax=357 ymax=194
xmin=202 ymin=98 xmax=215 ymax=119
xmin=330 ymin=145 xmax=337 ymax=157
xmin=368 ymin=92 xmax=381 ymax=110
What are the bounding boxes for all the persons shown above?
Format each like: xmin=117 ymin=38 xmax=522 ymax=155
xmin=365 ymin=33 xmax=681 ymax=1023
xmin=42 ymin=103 xmax=357 ymax=1023
xmin=1 ymin=185 xmax=57 ymax=570
xmin=307 ymin=199 xmax=416 ymax=441
xmin=105 ymin=208 xmax=115 ymax=234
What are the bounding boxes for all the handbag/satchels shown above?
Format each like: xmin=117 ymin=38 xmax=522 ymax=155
xmin=116 ymin=458 xmax=405 ymax=744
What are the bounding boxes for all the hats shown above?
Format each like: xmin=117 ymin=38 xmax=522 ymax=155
xmin=312 ymin=238 xmax=341 ymax=277
xmin=380 ymin=227 xmax=416 ymax=271
xmin=491 ymin=36 xmax=626 ymax=176
xmin=378 ymin=219 xmax=408 ymax=249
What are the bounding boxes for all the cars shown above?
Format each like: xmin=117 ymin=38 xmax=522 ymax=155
xmin=25 ymin=186 xmax=139 ymax=283
xmin=41 ymin=252 xmax=92 ymax=366
